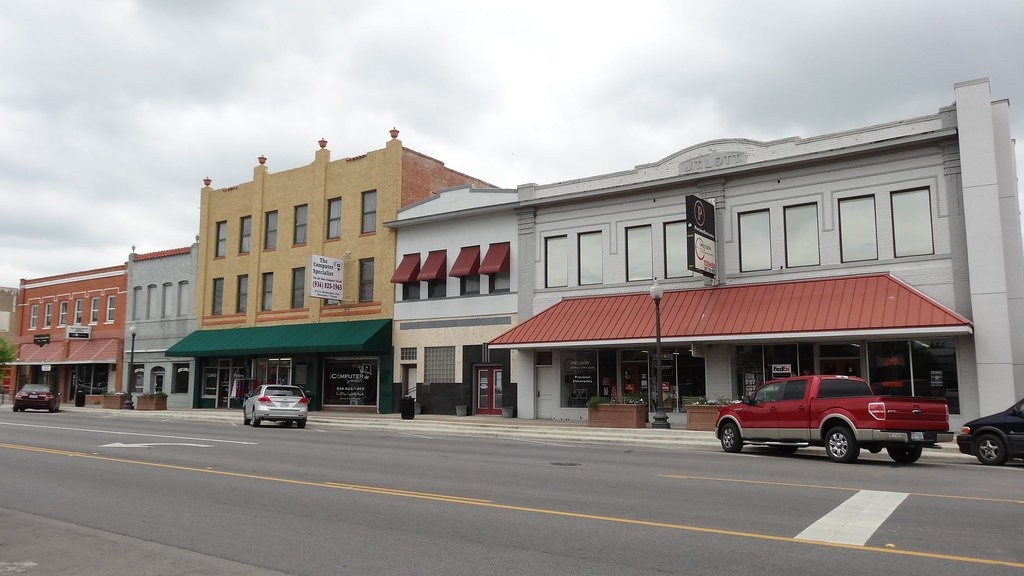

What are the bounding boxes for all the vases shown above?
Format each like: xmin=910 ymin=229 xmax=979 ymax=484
xmin=102 ymin=395 xmax=126 ymax=409
xmin=588 ymin=404 xmax=646 ymax=428
xmin=685 ymin=405 xmax=719 ymax=431
xmin=136 ymin=396 xmax=167 ymax=411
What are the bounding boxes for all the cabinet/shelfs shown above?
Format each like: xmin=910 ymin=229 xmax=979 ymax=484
xmin=736 ymin=366 xmax=768 ymax=398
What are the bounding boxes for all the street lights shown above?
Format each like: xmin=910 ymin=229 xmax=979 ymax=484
xmin=121 ymin=323 xmax=138 ymax=410
xmin=649 ymin=279 xmax=671 ymax=429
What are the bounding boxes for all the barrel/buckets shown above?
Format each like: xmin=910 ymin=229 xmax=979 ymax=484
xmin=414 ymin=402 xmax=422 ymax=415
xmin=501 ymin=406 xmax=514 ymax=418
xmin=455 ymin=405 xmax=467 ymax=416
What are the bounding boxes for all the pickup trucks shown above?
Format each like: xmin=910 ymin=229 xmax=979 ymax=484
xmin=714 ymin=373 xmax=955 ymax=464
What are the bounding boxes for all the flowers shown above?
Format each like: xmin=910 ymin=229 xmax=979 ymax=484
xmin=140 ymin=391 xmax=168 ymax=399
xmin=104 ymin=390 xmax=125 ymax=396
xmin=585 ymin=393 xmax=645 ymax=407
xmin=690 ymin=392 xmax=744 ymax=406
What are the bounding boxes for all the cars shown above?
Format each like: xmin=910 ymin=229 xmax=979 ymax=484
xmin=243 ymin=384 xmax=309 ymax=429
xmin=13 ymin=383 xmax=62 ymax=413
xmin=958 ymin=398 xmax=1024 ymax=467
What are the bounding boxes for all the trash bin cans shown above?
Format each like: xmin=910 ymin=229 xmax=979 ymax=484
xmin=75 ymin=392 xmax=85 ymax=407
xmin=400 ymin=397 xmax=416 ymax=420
